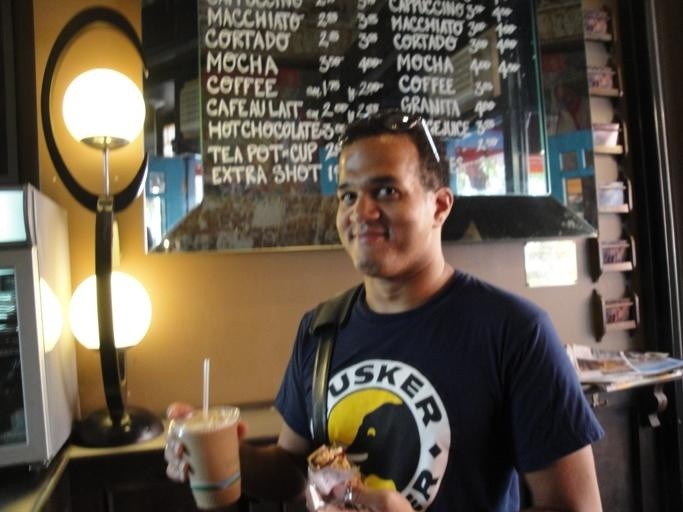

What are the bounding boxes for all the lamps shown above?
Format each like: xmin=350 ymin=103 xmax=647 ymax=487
xmin=39 ymin=5 xmax=166 ymax=449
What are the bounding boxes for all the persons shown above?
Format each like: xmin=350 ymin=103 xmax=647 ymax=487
xmin=163 ymin=108 xmax=606 ymax=510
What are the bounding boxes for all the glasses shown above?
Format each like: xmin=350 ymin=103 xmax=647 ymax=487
xmin=338 ymin=113 xmax=444 ymax=174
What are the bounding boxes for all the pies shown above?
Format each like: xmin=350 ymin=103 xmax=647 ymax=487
xmin=306 ymin=443 xmax=364 ymax=504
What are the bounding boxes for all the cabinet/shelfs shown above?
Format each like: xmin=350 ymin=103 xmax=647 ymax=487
xmin=576 ymin=5 xmax=643 ymax=343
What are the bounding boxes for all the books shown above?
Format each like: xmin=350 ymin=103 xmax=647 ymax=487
xmin=563 ymin=341 xmax=682 ymax=395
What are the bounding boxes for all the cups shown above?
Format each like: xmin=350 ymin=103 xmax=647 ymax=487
xmin=174 ymin=405 xmax=243 ymax=507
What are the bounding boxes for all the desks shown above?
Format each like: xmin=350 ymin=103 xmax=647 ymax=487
xmin=67 ymin=380 xmax=672 ymax=512
